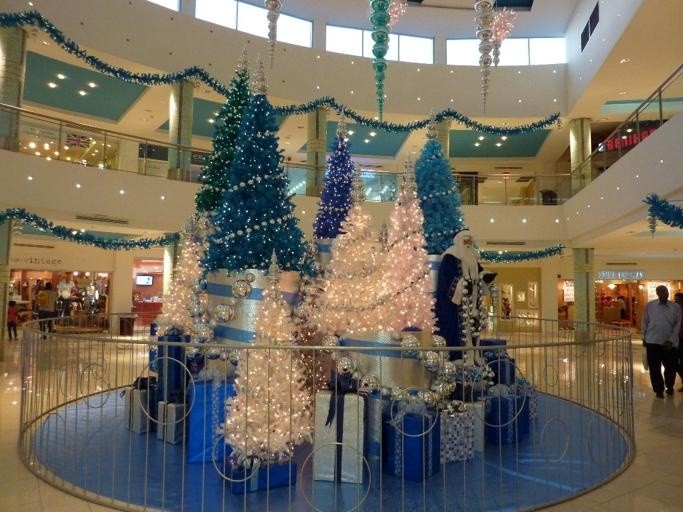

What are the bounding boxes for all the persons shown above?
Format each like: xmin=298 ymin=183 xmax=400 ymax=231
xmin=39 ymin=282 xmax=56 ymax=339
xmin=641 ymin=285 xmax=681 ymax=398
xmin=673 ymin=292 xmax=682 ymax=393
xmin=616 ymin=296 xmax=626 ymax=319
xmin=54 ymin=277 xmax=99 ymax=325
xmin=603 ymin=296 xmax=612 ymax=306
xmin=31 ymin=279 xmax=45 ymax=320
xmin=7 ymin=301 xmax=18 ymax=341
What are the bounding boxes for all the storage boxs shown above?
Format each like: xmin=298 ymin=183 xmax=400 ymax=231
xmin=119 ymin=326 xmax=298 ymax=496
xmin=311 ymin=339 xmax=539 ymax=484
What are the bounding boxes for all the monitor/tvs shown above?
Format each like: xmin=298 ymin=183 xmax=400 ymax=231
xmin=136 ymin=274 xmax=153 ymax=286
